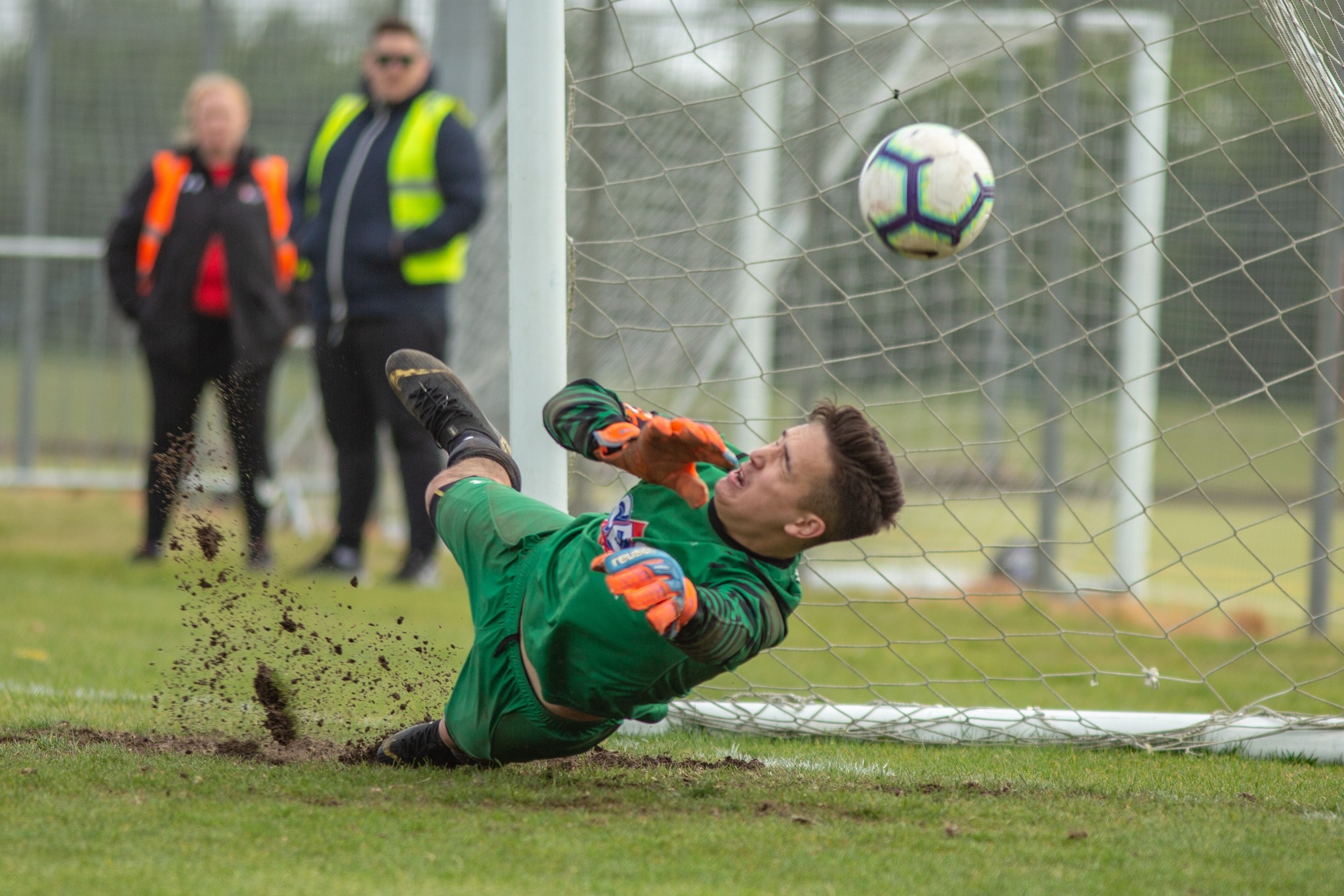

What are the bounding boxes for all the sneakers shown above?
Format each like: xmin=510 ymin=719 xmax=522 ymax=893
xmin=376 ymin=720 xmax=460 ymax=769
xmin=384 ymin=347 xmax=514 ymax=456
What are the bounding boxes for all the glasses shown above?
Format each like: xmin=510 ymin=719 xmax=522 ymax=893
xmin=375 ymin=54 xmax=413 ymax=68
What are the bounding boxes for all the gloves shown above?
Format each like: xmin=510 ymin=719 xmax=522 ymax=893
xmin=593 ymin=546 xmax=699 ymax=642
xmin=591 ymin=415 xmax=738 ymax=510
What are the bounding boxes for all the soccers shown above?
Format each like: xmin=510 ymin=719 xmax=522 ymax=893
xmin=858 ymin=122 xmax=993 ymax=259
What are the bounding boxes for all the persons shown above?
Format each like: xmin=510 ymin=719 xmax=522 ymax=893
xmin=288 ymin=21 xmax=487 ymax=586
xmin=371 ymin=350 xmax=904 ymax=770
xmin=101 ymin=71 xmax=297 ymax=571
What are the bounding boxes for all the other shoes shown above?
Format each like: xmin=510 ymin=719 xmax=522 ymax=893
xmin=295 ymin=544 xmax=362 ymax=580
xmin=130 ymin=540 xmax=160 ymax=565
xmin=393 ymin=552 xmax=435 ymax=588
xmin=246 ymin=550 xmax=271 ymax=574
